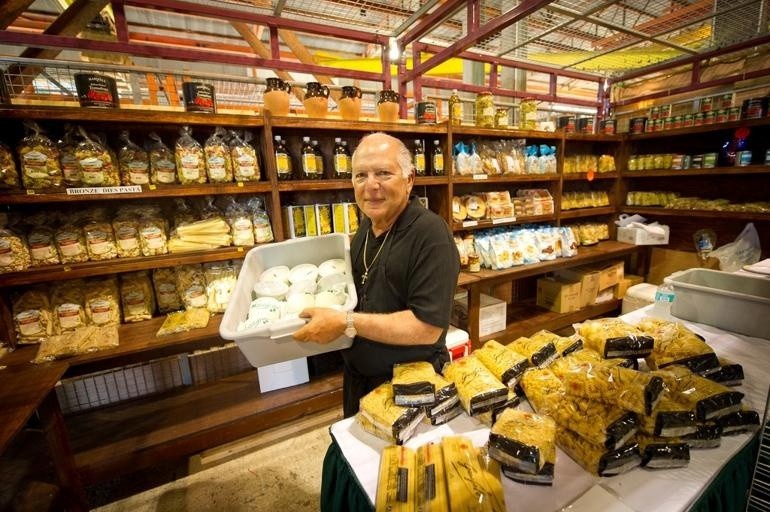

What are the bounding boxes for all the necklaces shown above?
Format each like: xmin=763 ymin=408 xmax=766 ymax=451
xmin=361 ymin=224 xmax=392 ymax=285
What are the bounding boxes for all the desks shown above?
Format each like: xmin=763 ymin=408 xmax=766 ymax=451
xmin=320 ymin=302 xmax=770 ymax=511
xmin=0 ymin=362 xmax=89 ymax=512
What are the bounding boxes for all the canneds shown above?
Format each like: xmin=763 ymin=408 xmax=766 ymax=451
xmin=735 ymin=151 xmax=752 ymax=167
xmin=672 ymin=153 xmax=718 ymax=170
xmin=74 ymin=72 xmax=120 ymax=109
xmin=415 ymin=103 xmax=436 ymax=126
xmin=643 ymin=93 xmax=741 ymax=133
xmin=765 ymin=151 xmax=770 ymax=164
xmin=740 ymin=98 xmax=770 ymax=119
xmin=183 ymin=82 xmax=217 ymax=114
xmin=629 ymin=117 xmax=647 ymax=132
xmin=557 ymin=115 xmax=593 ymax=133
xmin=599 ymin=120 xmax=617 ymax=134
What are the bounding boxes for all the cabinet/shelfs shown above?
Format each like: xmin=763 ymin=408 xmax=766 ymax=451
xmin=0 ymin=102 xmax=277 ymax=422
xmin=267 ymin=110 xmax=449 ymax=403
xmin=449 ymin=120 xmax=646 ymax=351
xmin=616 ymin=116 xmax=770 ymax=277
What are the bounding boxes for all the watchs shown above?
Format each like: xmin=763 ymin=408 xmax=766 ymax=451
xmin=344 ymin=311 xmax=357 ymax=338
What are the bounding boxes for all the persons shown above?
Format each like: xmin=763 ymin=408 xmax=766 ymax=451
xmin=292 ymin=133 xmax=460 ymax=419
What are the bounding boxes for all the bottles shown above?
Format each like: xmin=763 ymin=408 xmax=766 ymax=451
xmin=301 ymin=136 xmax=324 ymax=180
xmin=332 ymin=137 xmax=353 ymax=179
xmin=430 ymin=140 xmax=444 ymax=177
xmin=651 ymin=276 xmax=674 ymax=322
xmin=468 ymin=252 xmax=482 ymax=273
xmin=413 ymin=139 xmax=427 ymax=175
xmin=692 ymin=228 xmax=718 ymax=260
xmin=272 ymin=134 xmax=297 ymax=180
xmin=449 ymin=88 xmax=463 ymax=126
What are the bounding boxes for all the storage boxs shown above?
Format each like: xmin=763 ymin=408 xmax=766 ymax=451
xmin=453 ymin=291 xmax=507 ymax=338
xmin=257 ymin=356 xmax=317 ymax=394
xmin=219 ymin=232 xmax=359 ymax=369
xmin=535 ymin=259 xmax=644 ymax=314
xmin=663 ymin=268 xmax=770 ymax=341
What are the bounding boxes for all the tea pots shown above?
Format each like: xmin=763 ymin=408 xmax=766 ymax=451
xmin=337 ymin=85 xmax=363 ymax=119
xmin=302 ymin=80 xmax=331 ymax=119
xmin=376 ymin=89 xmax=401 ymax=123
xmin=262 ymin=75 xmax=293 ymax=115
